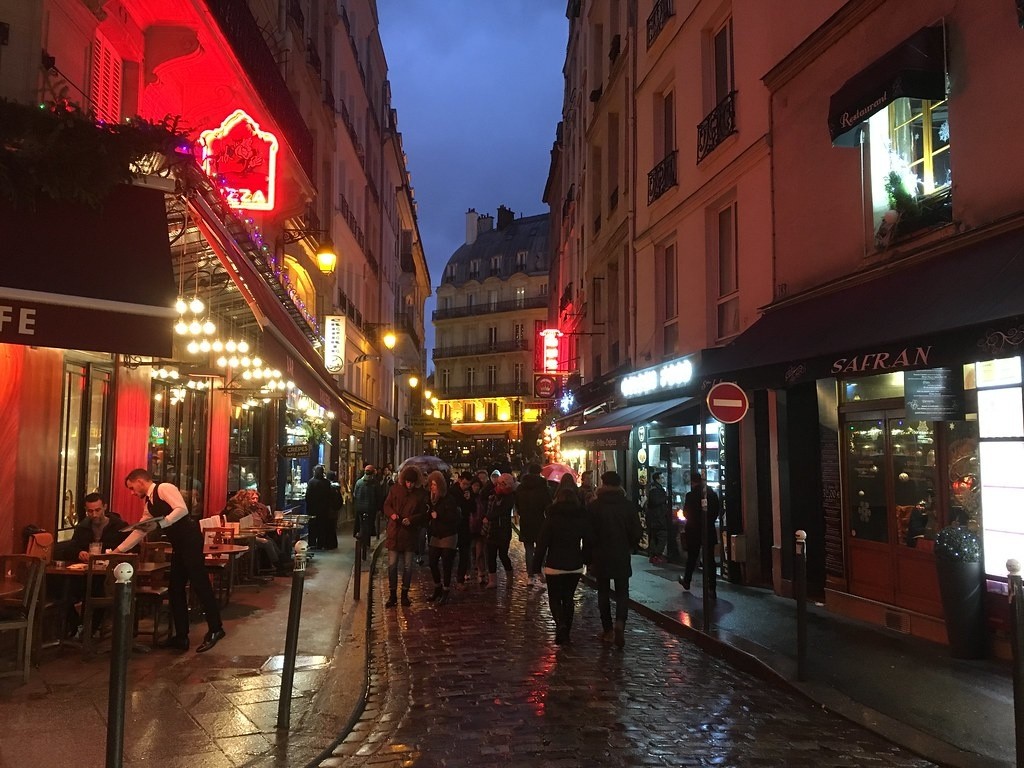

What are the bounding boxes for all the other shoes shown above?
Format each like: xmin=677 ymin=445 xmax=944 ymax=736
xmin=649 ymin=555 xmax=663 ymax=565
xmin=554 ymin=622 xmax=572 ymax=646
xmin=455 ymin=571 xmax=487 ymax=591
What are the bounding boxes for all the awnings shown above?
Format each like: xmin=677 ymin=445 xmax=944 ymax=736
xmin=691 ymin=228 xmax=1024 ymax=375
xmin=554 ymin=396 xmax=703 ymax=438
xmin=175 ymin=192 xmax=360 ymax=419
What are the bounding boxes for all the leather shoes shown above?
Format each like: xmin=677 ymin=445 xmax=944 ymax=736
xmin=157 ymin=637 xmax=189 ymax=650
xmin=196 ymin=628 xmax=225 ymax=653
xmin=67 ymin=623 xmax=83 ymax=642
xmin=678 ymin=573 xmax=690 ymax=590
xmin=92 ymin=619 xmax=103 ymax=639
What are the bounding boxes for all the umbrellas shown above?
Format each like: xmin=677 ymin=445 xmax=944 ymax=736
xmin=540 ymin=462 xmax=579 ymax=484
xmin=396 ymin=454 xmax=449 ymax=475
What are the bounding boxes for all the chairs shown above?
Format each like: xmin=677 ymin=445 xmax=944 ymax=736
xmin=18 ymin=554 xmax=64 ymax=658
xmin=199 ymin=505 xmax=284 ymax=586
xmin=202 ymin=527 xmax=235 ymax=609
xmin=133 ymin=541 xmax=172 ymax=646
xmin=0 ymin=556 xmax=46 ymax=683
xmin=83 ymin=553 xmax=137 ymax=647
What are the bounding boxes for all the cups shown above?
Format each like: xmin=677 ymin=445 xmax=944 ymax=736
xmin=89 ymin=542 xmax=102 ymax=554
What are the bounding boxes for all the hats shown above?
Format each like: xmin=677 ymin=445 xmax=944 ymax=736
xmin=364 ymin=464 xmax=375 ymax=471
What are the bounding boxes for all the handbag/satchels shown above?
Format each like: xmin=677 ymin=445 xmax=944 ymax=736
xmin=22 ymin=524 xmax=53 ymax=567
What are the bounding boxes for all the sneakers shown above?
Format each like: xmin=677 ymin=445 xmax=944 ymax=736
xmin=526 ymin=575 xmax=541 ymax=587
xmin=595 ymin=623 xmax=626 ymax=651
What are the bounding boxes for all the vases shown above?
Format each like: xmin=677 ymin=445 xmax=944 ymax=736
xmin=936 ymin=559 xmax=987 ymax=659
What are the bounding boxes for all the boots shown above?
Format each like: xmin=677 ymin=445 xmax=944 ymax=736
xmin=485 ymin=573 xmax=497 ymax=589
xmin=505 ymin=570 xmax=514 ymax=589
xmin=384 ymin=566 xmax=398 ymax=610
xmin=426 ymin=584 xmax=451 ymax=606
xmin=400 ymin=566 xmax=411 ymax=606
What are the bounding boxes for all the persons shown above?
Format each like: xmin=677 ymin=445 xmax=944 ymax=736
xmin=305 ymin=465 xmax=343 ymax=550
xmin=645 ymin=471 xmax=671 ymax=564
xmin=677 ymin=473 xmax=722 ymax=590
xmin=109 ymin=467 xmax=226 ymax=654
xmin=61 ymin=493 xmax=141 ymax=637
xmin=222 ymin=489 xmax=297 ymax=577
xmin=354 ymin=453 xmax=515 ymax=607
xmin=516 ymin=464 xmax=644 ymax=647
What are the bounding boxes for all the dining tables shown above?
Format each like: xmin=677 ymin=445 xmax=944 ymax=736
xmin=208 ymin=514 xmax=318 ymax=585
xmin=45 ymin=559 xmax=171 ymax=640
xmin=0 ymin=581 xmax=24 ymax=596
xmin=155 ymin=544 xmax=249 ymax=613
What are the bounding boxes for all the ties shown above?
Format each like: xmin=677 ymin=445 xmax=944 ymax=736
xmin=145 ymin=497 xmax=153 ymax=509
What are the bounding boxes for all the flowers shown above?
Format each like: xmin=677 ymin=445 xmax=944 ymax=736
xmin=934 ymin=524 xmax=981 ymax=563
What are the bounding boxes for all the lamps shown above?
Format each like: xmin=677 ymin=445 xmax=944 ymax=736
xmin=317 ymin=241 xmax=337 ymax=277
